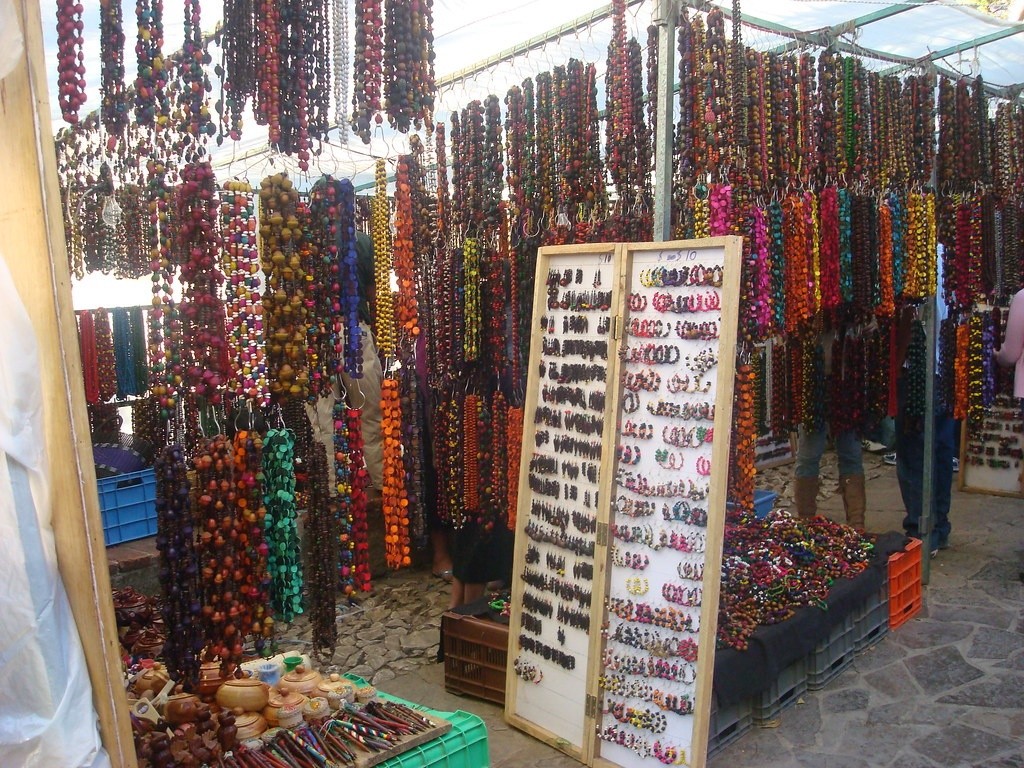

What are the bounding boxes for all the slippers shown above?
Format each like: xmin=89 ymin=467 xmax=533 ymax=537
xmin=431 ymin=567 xmax=455 ymax=584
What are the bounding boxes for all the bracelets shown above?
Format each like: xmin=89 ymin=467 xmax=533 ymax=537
xmin=718 ymin=509 xmax=878 ymax=653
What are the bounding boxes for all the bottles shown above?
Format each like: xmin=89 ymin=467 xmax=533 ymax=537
xmin=283 ymin=657 xmax=303 ymax=673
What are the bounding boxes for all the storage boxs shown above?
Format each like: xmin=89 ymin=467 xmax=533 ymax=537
xmin=441 ymin=490 xmax=924 ymax=762
xmin=96 ymin=465 xmax=158 ymax=547
xmin=341 ymin=671 xmax=491 ymax=768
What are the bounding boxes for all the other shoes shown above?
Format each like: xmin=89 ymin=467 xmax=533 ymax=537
xmin=862 ymin=440 xmax=887 ymax=451
xmin=883 ymin=449 xmax=896 ymax=465
xmin=952 ymin=457 xmax=961 ymax=473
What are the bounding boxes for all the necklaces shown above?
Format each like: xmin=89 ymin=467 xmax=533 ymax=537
xmin=418 ymin=0 xmax=1024 ymax=531
xmin=51 ymin=1 xmax=516 ymax=678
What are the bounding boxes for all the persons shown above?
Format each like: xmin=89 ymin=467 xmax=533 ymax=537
xmin=890 ymin=298 xmax=957 ymax=554
xmin=790 ymin=311 xmax=879 ymax=532
